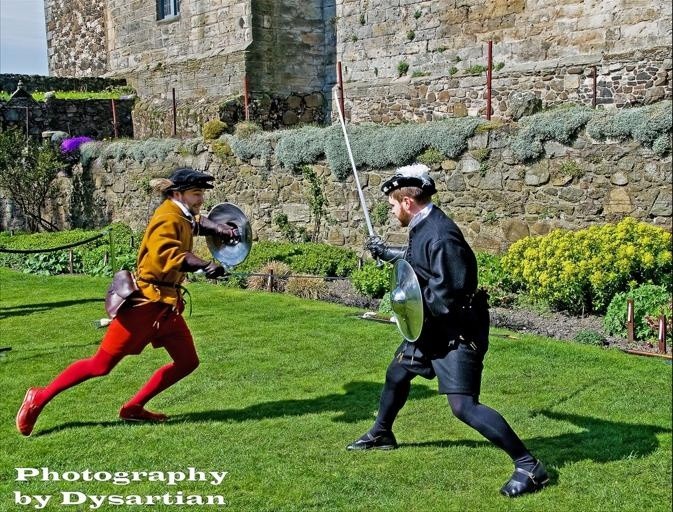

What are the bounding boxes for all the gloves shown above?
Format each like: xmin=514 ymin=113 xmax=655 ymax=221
xmin=195 ymin=213 xmax=242 ymax=249
xmin=363 ymin=237 xmax=411 ymax=266
xmin=176 ymin=250 xmax=227 ymax=281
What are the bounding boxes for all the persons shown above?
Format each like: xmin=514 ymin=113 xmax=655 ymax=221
xmin=15 ymin=168 xmax=238 ymax=436
xmin=345 ymin=165 xmax=550 ymax=497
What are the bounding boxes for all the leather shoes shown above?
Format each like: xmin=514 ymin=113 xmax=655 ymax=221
xmin=13 ymin=384 xmax=48 ymax=438
xmin=346 ymin=426 xmax=403 ymax=456
xmin=117 ymin=401 xmax=169 ymax=426
xmin=496 ymin=456 xmax=554 ymax=500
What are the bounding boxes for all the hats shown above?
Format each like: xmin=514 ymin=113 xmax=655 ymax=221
xmin=146 ymin=164 xmax=217 ymax=198
xmin=378 ymin=163 xmax=438 ymax=199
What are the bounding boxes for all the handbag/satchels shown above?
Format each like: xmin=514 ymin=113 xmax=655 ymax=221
xmin=102 ymin=268 xmax=141 ymax=320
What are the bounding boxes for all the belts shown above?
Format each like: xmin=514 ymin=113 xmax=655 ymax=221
xmin=134 ymin=273 xmax=197 ymax=317
